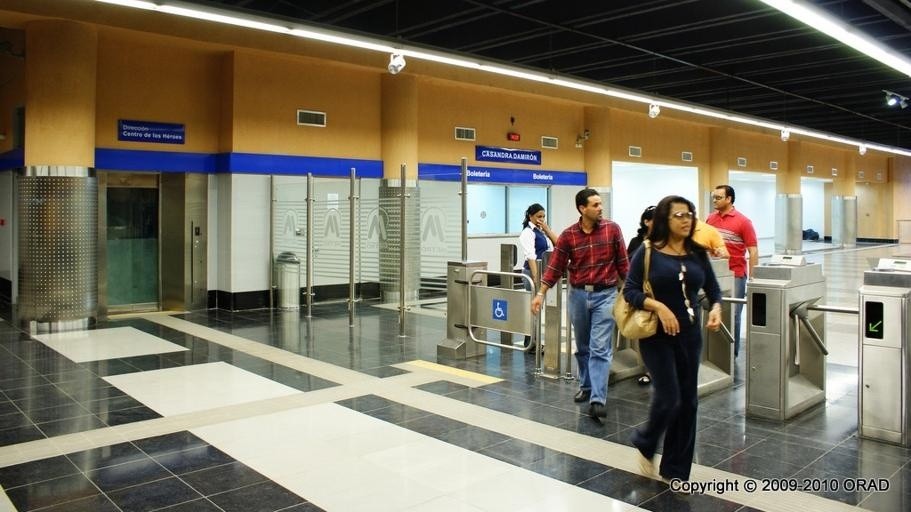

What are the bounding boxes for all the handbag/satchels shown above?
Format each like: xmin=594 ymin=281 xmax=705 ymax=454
xmin=537 ymin=293 xmax=544 ymax=296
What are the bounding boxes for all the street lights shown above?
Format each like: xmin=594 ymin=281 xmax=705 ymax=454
xmin=612 ymin=238 xmax=660 ymax=340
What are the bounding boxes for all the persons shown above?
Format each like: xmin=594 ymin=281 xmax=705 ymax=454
xmin=532 ymin=188 xmax=629 ymax=426
xmin=690 ymin=219 xmax=729 ymax=260
xmin=705 ymin=185 xmax=758 ymax=357
xmin=629 ymin=206 xmax=655 ymax=384
xmin=624 ymin=197 xmax=722 ymax=493
xmin=513 ymin=204 xmax=560 ymax=354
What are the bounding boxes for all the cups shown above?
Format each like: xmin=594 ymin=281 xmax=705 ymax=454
xmin=713 ymin=195 xmax=726 ymax=201
xmin=668 ymin=211 xmax=693 ymax=221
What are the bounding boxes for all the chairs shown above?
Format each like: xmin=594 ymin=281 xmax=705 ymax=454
xmin=529 ymin=343 xmax=544 ymax=354
xmin=588 ymin=402 xmax=606 ymax=418
xmin=632 ymin=445 xmax=656 ymax=478
xmin=638 ymin=371 xmax=651 ymax=384
xmin=659 ymin=475 xmax=691 ymax=494
xmin=574 ymin=389 xmax=589 ymax=403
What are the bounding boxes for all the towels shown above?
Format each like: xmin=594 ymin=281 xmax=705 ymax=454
xmin=275 ymin=252 xmax=300 ymax=308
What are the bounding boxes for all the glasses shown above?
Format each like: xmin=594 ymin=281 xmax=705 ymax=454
xmin=758 ymin=0 xmax=911 ymax=78
xmin=388 ymin=56 xmax=406 ymax=75
xmin=648 ymin=106 xmax=660 ymax=118
xmin=882 ymin=90 xmax=909 ymax=109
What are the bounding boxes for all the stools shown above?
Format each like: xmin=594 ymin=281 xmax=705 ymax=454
xmin=572 ymin=282 xmax=612 ymax=292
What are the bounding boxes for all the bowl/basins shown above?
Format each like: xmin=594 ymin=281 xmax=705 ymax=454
xmin=666 ymin=243 xmax=685 ymax=256
